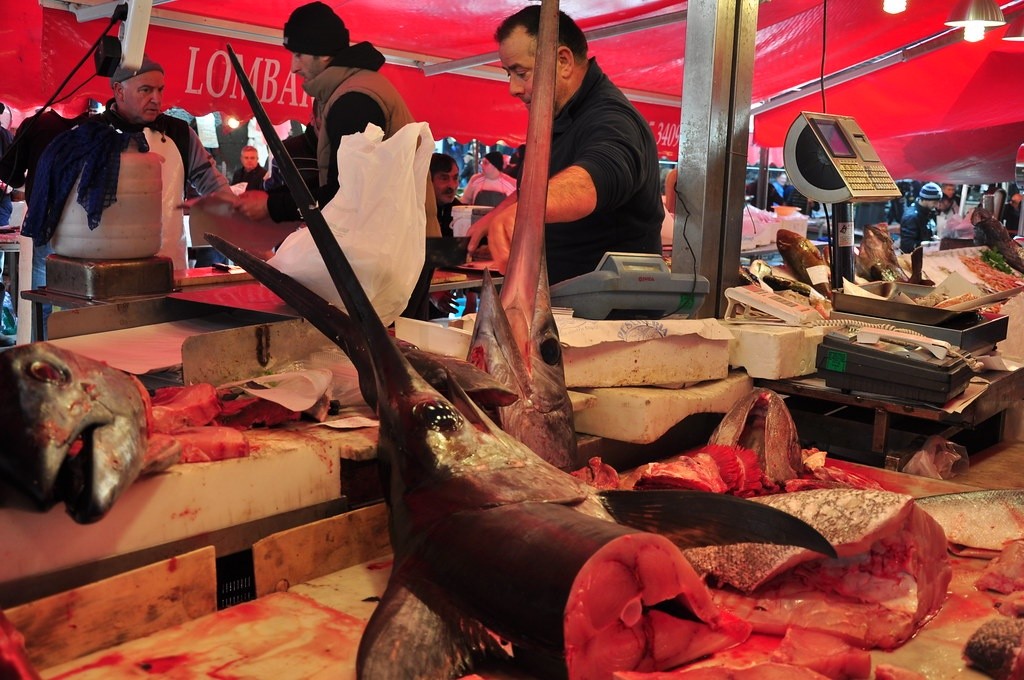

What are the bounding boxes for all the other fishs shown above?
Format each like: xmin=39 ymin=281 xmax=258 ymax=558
xmin=971 ymin=208 xmax=1024 ymax=274
xmin=774 ymin=228 xmax=832 ymax=298
xmin=1 ymin=342 xmax=148 ymax=525
xmin=824 ymin=225 xmax=934 ymax=286
xmin=738 ymin=259 xmax=833 ymax=319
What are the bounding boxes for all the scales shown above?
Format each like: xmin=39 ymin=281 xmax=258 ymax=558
xmin=781 ymin=110 xmax=1012 ymax=361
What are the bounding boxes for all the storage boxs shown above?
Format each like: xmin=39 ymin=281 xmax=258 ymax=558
xmin=718 ymin=318 xmax=846 ymax=380
xmin=741 ymin=218 xmax=808 ymax=251
xmin=462 ymin=315 xmax=731 ymax=389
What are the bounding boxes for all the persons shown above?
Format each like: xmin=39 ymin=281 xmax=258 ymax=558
xmin=746 ymin=167 xmax=820 ymax=219
xmin=467 ymin=5 xmax=664 ymax=285
xmin=460 ymin=151 xmax=517 ymax=207
xmin=855 ymin=180 xmax=1022 ymax=257
xmin=428 ymin=153 xmax=476 ymax=315
xmin=81 ymin=49 xmax=233 ymax=271
xmin=503 ymin=143 xmax=525 ymax=178
xmin=0 ymin=102 xmax=324 ymax=345
xmin=282 ymin=2 xmax=443 ymax=326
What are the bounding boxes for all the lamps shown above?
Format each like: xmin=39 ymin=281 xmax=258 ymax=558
xmin=944 ymin=0 xmax=1007 ymax=43
xmin=1002 ymin=9 xmax=1024 ymax=42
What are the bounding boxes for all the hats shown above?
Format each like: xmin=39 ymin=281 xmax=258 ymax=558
xmin=283 ymin=1 xmax=349 ymax=57
xmin=111 ymin=52 xmax=164 ymax=89
xmin=919 ymin=182 xmax=943 ymax=201
xmin=484 ymin=151 xmax=503 ymax=171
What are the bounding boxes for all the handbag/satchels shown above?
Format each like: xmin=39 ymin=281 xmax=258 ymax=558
xmin=944 ymin=208 xmax=974 ymax=240
xmin=266 ymin=122 xmax=435 ymax=328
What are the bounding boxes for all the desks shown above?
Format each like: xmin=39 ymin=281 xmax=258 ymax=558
xmin=741 ymin=238 xmax=829 ymax=266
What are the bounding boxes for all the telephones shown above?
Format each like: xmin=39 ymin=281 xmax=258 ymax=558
xmin=548 ymin=251 xmax=712 ymax=321
xmin=812 ymin=326 xmax=977 ymax=407
